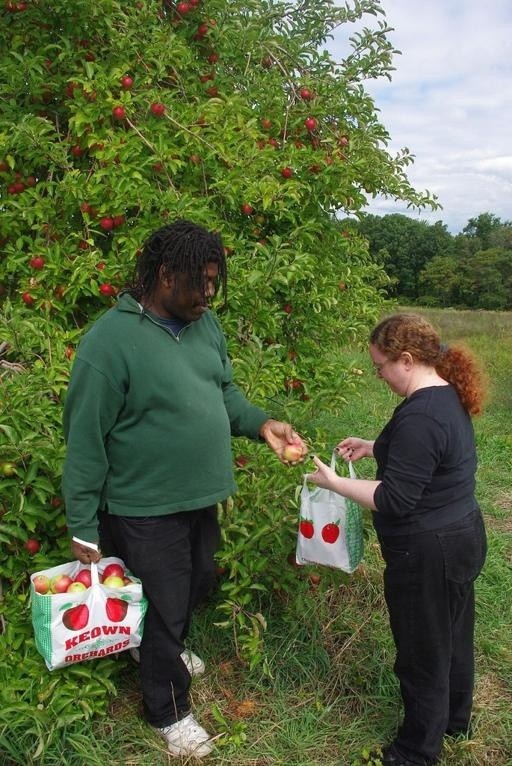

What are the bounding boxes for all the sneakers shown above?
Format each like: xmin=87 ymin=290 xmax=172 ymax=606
xmin=361 ymin=730 xmax=472 ymax=766
xmin=146 ymin=711 xmax=215 ymax=757
xmin=129 ymin=645 xmax=206 ymax=679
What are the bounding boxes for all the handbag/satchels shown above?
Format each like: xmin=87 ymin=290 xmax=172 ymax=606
xmin=29 ymin=535 xmax=150 ymax=672
xmin=295 ymin=446 xmax=366 ymax=574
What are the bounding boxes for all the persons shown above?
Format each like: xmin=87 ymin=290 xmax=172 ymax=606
xmin=60 ymin=218 xmax=309 ymax=765
xmin=299 ymin=313 xmax=493 ymax=763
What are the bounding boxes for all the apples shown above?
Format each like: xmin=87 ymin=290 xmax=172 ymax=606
xmin=58 ymin=601 xmax=89 ymax=630
xmin=1 ymin=0 xmax=365 ymax=551
xmin=105 ymin=595 xmax=132 ymax=622
xmin=32 ymin=563 xmax=127 ymax=596
